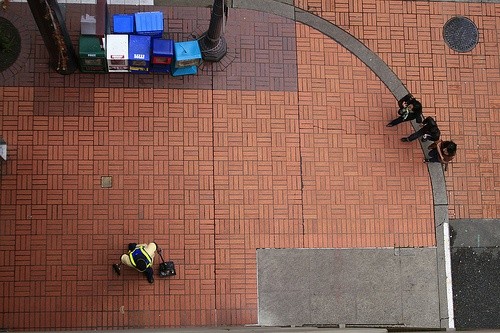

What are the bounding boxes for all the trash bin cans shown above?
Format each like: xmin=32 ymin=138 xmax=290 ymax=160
xmin=172 ymin=41 xmax=200 ymax=77
xmin=106 ymin=35 xmax=130 ymax=74
xmin=153 ymin=38 xmax=174 ymax=73
xmin=135 ymin=10 xmax=163 ymax=36
xmin=79 ymin=32 xmax=107 ymax=73
xmin=130 ymin=35 xmax=152 ymax=72
xmin=112 ymin=13 xmax=135 ymax=34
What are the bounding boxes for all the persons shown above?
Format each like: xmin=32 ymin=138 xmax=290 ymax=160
xmin=386 ymin=95 xmax=422 ymax=127
xmin=423 ymin=140 xmax=457 ymax=163
xmin=120 ymin=242 xmax=160 ymax=283
xmin=401 ymin=116 xmax=440 ymax=142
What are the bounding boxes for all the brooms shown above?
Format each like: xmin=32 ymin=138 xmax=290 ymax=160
xmin=112 ymin=262 xmax=123 ymax=275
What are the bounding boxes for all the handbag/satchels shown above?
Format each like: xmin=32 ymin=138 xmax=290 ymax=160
xmin=397 ymin=93 xmax=413 ymax=108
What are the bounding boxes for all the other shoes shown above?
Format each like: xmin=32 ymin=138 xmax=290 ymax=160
xmin=400 ymin=138 xmax=408 ymax=142
xmin=146 ymin=274 xmax=154 ymax=283
xmin=386 ymin=123 xmax=393 ymax=127
xmin=425 ymin=159 xmax=430 ymax=162
